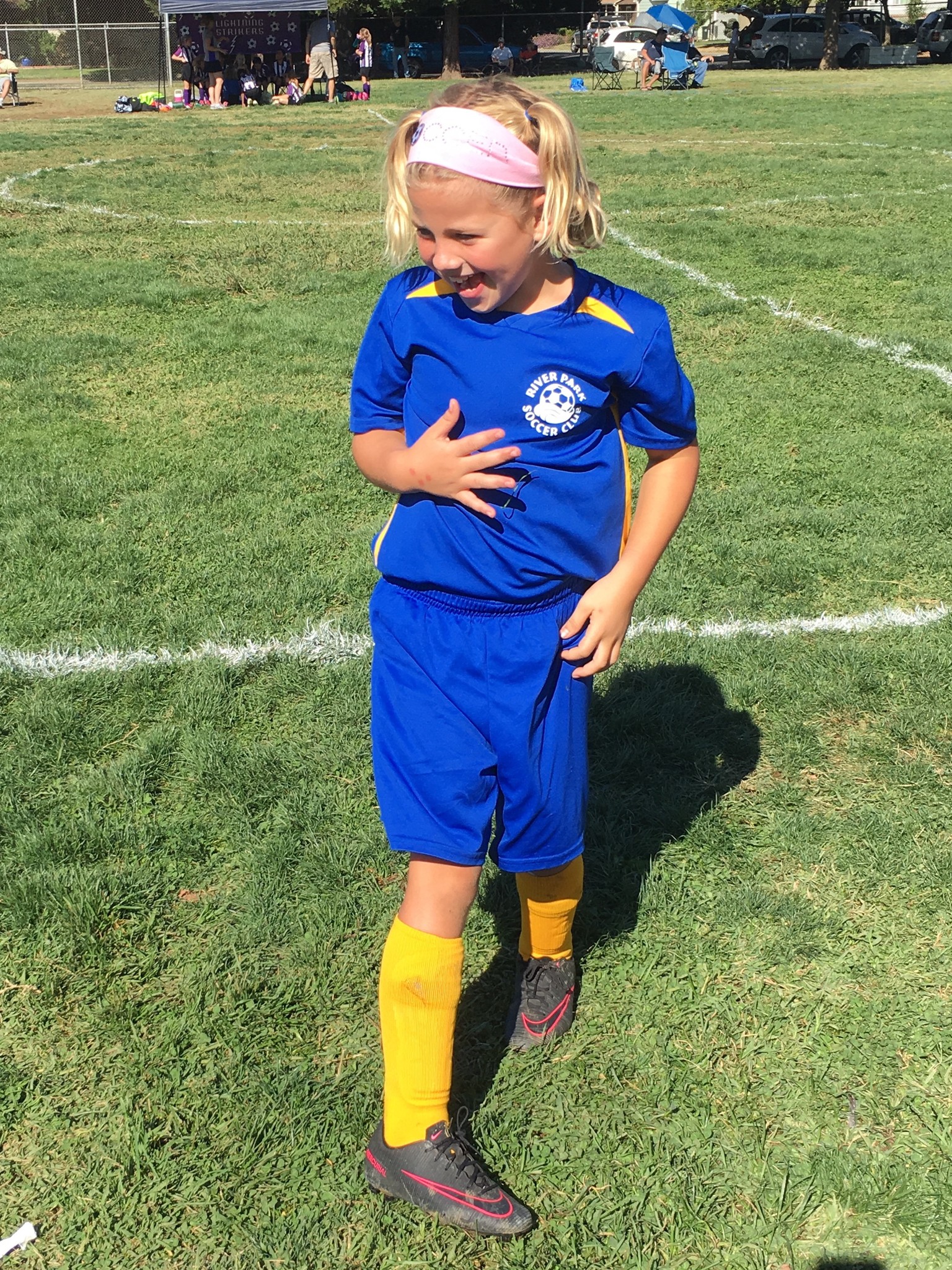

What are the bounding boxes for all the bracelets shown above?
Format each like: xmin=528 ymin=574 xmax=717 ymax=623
xmin=306 ymin=53 xmax=309 ymax=55
xmin=333 ymin=47 xmax=336 ymax=49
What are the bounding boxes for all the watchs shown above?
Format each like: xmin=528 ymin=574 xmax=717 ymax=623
xmin=5 ymin=69 xmax=7 ymax=73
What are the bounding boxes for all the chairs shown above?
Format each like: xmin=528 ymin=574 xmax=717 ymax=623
xmin=662 ymin=41 xmax=691 ymax=89
xmin=636 ymin=51 xmax=662 ymax=90
xmin=592 ymin=45 xmax=625 ymax=90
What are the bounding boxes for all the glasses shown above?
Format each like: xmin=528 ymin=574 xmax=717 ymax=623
xmin=683 ymin=39 xmax=690 ymax=41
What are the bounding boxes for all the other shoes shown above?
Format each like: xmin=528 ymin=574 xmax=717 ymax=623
xmin=210 ymin=104 xmax=224 ymax=109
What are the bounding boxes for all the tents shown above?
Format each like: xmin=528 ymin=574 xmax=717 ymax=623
xmin=157 ymin=0 xmax=333 ymax=100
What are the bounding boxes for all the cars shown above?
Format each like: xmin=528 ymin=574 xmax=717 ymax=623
xmin=385 ymin=16 xmax=538 ymax=78
xmin=836 ymin=9 xmax=915 ymax=47
xmin=593 ymin=25 xmax=660 ymax=69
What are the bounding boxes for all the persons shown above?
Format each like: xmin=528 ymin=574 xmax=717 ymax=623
xmin=390 ymin=15 xmax=412 ymax=79
xmin=171 ymin=33 xmax=196 ymax=109
xmin=195 ymin=59 xmax=211 ymax=106
xmin=680 ymin=33 xmax=715 ymax=89
xmin=727 ymin=21 xmax=748 ymax=70
xmin=222 ymin=65 xmax=243 ymax=106
xmin=491 ymin=36 xmax=514 ymax=76
xmin=199 ymin=14 xmax=230 ymax=110
xmin=355 ymin=27 xmax=374 ymax=100
xmin=251 ymin=56 xmax=272 ymax=92
xmin=270 ymin=72 xmax=302 ymax=106
xmin=232 ymin=51 xmax=251 ymax=82
xmin=302 ymin=11 xmax=339 ymax=103
xmin=238 ymin=68 xmax=263 ymax=106
xmin=345 ymin=69 xmax=703 ymax=1241
xmin=639 ymin=29 xmax=668 ymax=91
xmin=271 ymin=48 xmax=291 ymax=97
xmin=520 ymin=43 xmax=538 ymax=77
xmin=0 ymin=46 xmax=19 ymax=107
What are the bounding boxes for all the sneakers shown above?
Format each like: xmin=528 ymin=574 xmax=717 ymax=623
xmin=503 ymin=949 xmax=576 ymax=1053
xmin=363 ymin=1120 xmax=535 ymax=1236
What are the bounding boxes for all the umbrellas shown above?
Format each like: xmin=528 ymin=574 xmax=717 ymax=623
xmin=646 ymin=3 xmax=698 ymax=34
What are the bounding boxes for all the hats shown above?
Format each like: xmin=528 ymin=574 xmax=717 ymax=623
xmin=394 ymin=16 xmax=401 ymax=24
xmin=498 ymin=37 xmax=504 ymax=43
xmin=0 ymin=46 xmax=6 ymax=55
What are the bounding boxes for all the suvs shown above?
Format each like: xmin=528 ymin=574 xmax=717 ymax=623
xmin=726 ymin=4 xmax=879 ymax=71
xmin=917 ymin=9 xmax=952 ymax=62
xmin=569 ymin=15 xmax=631 ymax=55
